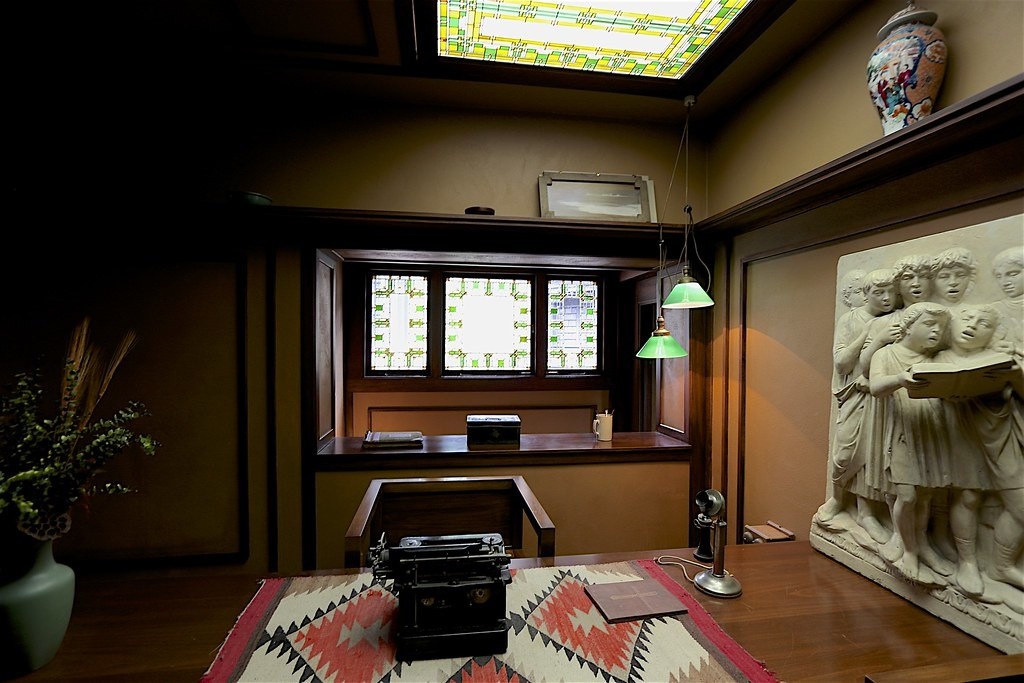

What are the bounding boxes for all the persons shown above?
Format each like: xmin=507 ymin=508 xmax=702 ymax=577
xmin=817 ymin=247 xmax=1024 ymax=597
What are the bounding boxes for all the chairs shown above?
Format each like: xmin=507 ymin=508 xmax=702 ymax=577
xmin=341 ymin=474 xmax=556 ymax=566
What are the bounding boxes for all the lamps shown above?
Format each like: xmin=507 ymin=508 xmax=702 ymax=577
xmin=662 ymin=224 xmax=715 ymax=310
xmin=636 ymin=230 xmax=689 ymax=358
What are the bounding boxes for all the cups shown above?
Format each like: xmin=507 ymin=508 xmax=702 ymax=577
xmin=592 ymin=414 xmax=613 ymax=441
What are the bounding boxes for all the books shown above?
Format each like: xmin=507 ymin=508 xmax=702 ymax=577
xmin=908 ymin=353 xmax=1012 ymax=398
xmin=363 ymin=431 xmax=423 ymax=449
xmin=584 ymin=579 xmax=689 ymax=623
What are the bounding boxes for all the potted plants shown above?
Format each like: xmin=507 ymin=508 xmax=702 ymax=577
xmin=0 ymin=315 xmax=161 ymax=677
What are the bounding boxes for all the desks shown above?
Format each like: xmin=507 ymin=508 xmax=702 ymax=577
xmin=203 ymin=538 xmax=1024 ymax=683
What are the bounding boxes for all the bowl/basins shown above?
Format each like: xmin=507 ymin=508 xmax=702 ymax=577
xmin=221 ymin=190 xmax=272 ymax=207
xmin=465 ymin=206 xmax=495 ymax=216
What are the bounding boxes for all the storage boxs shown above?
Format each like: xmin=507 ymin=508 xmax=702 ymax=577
xmin=467 ymin=415 xmax=521 ymax=451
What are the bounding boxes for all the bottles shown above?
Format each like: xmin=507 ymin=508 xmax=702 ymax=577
xmin=866 ymin=1 xmax=947 ymax=136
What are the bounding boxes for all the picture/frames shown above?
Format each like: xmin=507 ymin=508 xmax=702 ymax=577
xmin=536 ymin=172 xmax=650 ymax=222
